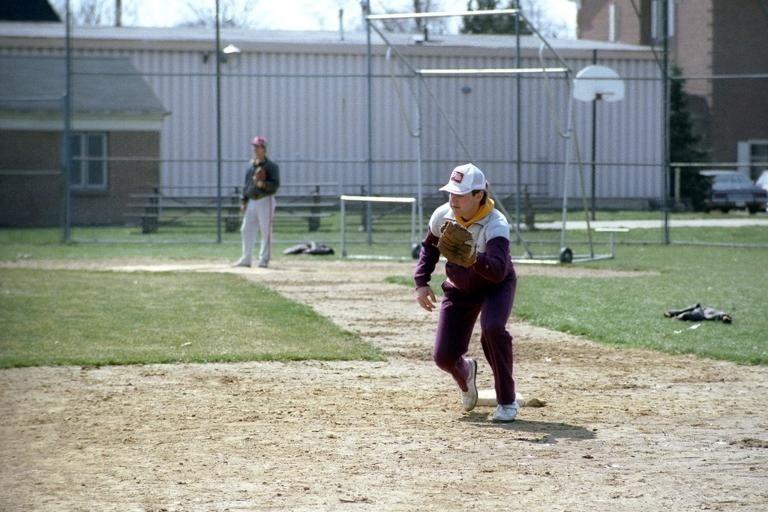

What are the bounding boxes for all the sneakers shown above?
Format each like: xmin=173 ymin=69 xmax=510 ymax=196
xmin=460 ymin=359 xmax=478 ymax=411
xmin=492 ymin=400 xmax=520 ymax=422
xmin=230 ymin=261 xmax=251 ymax=267
xmin=258 ymin=259 xmax=268 ymax=267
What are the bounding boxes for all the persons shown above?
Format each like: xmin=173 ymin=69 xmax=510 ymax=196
xmin=411 ymin=160 xmax=519 ymax=424
xmin=229 ymin=134 xmax=281 ymax=269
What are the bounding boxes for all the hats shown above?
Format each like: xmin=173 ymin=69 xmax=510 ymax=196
xmin=250 ymin=136 xmax=267 ymax=149
xmin=438 ymin=163 xmax=489 ymax=195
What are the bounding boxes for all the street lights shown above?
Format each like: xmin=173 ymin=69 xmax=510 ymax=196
xmin=202 ymin=43 xmax=241 ymax=243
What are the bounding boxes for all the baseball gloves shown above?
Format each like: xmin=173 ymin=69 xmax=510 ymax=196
xmin=437 ymin=221 xmax=478 ymax=268
xmin=252 ymin=167 xmax=265 ymax=185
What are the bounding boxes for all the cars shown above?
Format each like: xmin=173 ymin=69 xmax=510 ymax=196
xmin=690 ymin=169 xmax=767 ymax=215
xmin=753 ymin=170 xmax=767 ymax=213
